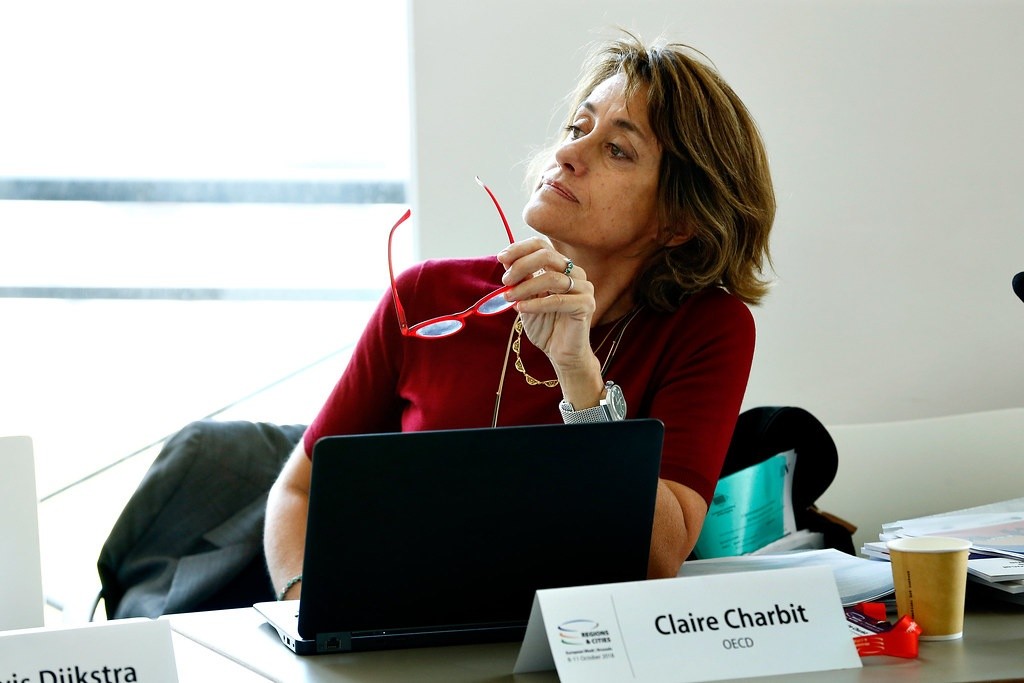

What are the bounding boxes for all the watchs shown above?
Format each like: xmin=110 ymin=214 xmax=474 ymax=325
xmin=559 ymin=385 xmax=627 ymax=422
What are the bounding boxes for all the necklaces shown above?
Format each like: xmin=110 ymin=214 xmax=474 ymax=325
xmin=513 ymin=289 xmax=631 ymax=387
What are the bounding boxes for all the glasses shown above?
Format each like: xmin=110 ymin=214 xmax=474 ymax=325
xmin=387 ymin=176 xmax=531 ymax=340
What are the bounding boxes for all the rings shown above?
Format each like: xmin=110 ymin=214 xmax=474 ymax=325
xmin=565 ymin=276 xmax=574 ymax=293
xmin=564 ymin=260 xmax=573 ymax=275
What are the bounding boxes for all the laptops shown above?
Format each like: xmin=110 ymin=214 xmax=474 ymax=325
xmin=253 ymin=417 xmax=666 ymax=657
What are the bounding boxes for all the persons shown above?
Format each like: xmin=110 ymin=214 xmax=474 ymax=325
xmin=264 ymin=41 xmax=774 ymax=600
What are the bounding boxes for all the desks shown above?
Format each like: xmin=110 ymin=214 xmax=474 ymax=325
xmin=1 ymin=607 xmax=1024 ymax=683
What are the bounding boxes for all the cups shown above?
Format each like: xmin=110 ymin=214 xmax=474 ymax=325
xmin=885 ymin=536 xmax=972 ymax=642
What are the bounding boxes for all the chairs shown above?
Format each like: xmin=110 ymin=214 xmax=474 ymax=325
xmin=0 ymin=420 xmax=308 ymax=631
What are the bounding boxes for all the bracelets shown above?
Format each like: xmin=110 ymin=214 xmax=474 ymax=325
xmin=276 ymin=575 xmax=302 ymax=600
